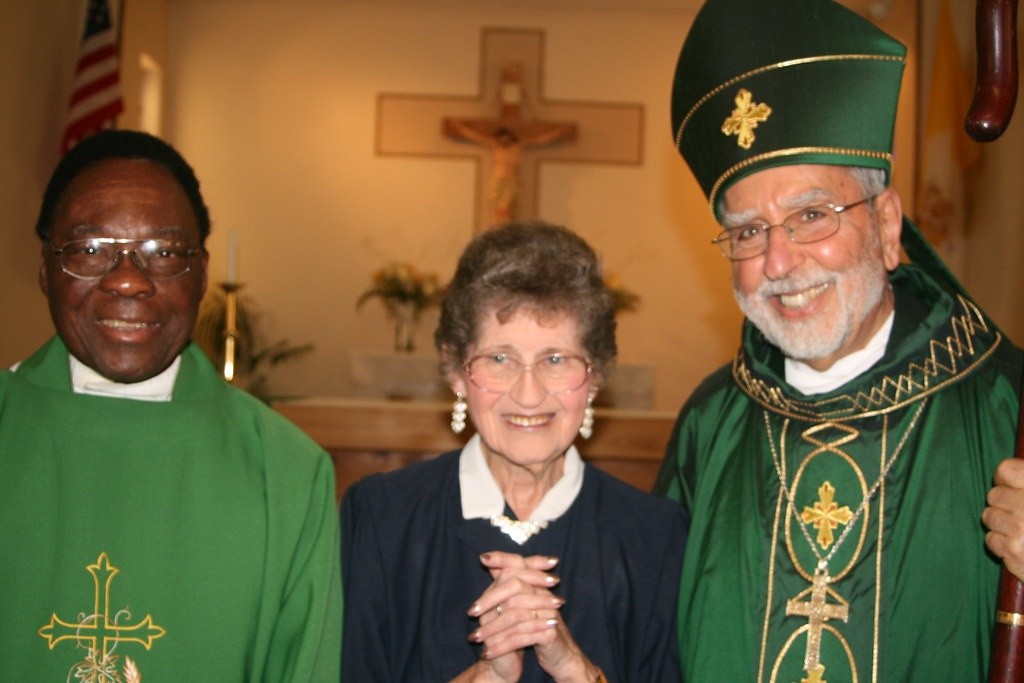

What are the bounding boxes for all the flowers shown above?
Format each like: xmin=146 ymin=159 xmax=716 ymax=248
xmin=354 ymin=261 xmax=448 ymax=315
xmin=600 ymin=270 xmax=645 ymax=314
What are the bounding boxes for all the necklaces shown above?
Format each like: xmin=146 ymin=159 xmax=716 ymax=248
xmin=763 ymin=398 xmax=927 ymax=669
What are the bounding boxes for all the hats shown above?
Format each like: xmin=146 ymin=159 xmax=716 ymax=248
xmin=668 ymin=0 xmax=907 ymax=226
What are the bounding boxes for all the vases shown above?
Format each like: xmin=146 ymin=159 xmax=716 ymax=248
xmin=389 ymin=302 xmax=421 ymax=403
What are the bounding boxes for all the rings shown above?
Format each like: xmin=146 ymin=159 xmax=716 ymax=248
xmin=496 ymin=605 xmax=503 ymax=615
xmin=531 ymin=610 xmax=537 ymax=619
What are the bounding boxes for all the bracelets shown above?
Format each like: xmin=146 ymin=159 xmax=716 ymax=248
xmin=595 ymin=667 xmax=607 ymax=683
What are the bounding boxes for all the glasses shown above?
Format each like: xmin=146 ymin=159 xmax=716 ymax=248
xmin=48 ymin=235 xmax=200 ymax=279
xmin=710 ymin=193 xmax=879 ymax=262
xmin=454 ymin=353 xmax=597 ymax=394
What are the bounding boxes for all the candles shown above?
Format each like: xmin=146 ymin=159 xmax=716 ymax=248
xmin=224 ymin=228 xmax=239 ymax=282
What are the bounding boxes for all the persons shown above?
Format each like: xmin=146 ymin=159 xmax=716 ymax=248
xmin=339 ymin=220 xmax=693 ymax=683
xmin=650 ymin=0 xmax=1024 ymax=683
xmin=0 ymin=130 xmax=342 ymax=683
